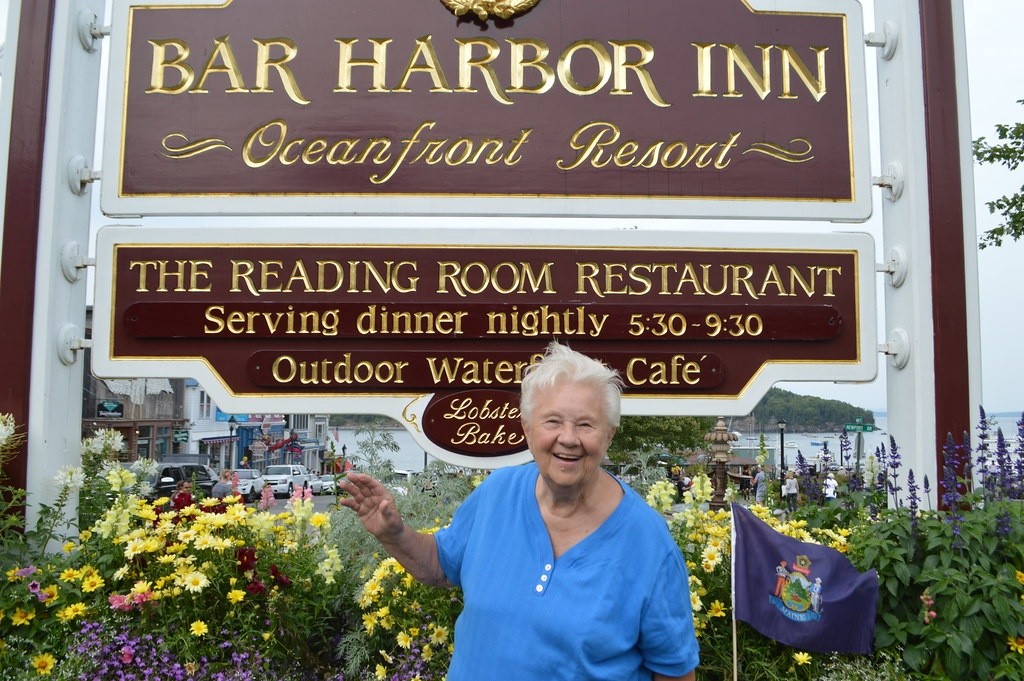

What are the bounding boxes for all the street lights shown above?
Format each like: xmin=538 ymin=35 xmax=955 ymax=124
xmin=224 ymin=414 xmax=239 ymax=473
xmin=776 ymin=419 xmax=786 ymax=501
xmin=342 ymin=444 xmax=347 ymax=481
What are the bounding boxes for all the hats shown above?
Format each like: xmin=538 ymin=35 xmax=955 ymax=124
xmin=828 ymin=473 xmax=834 ymax=478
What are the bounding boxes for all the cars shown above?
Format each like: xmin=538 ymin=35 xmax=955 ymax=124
xmin=307 ymin=473 xmax=324 ymax=497
xmin=203 ymin=465 xmax=219 ymax=485
xmin=218 ymin=469 xmax=265 ymax=502
xmin=646 ymin=453 xmax=688 ymax=468
xmin=319 ymin=475 xmax=342 ymax=495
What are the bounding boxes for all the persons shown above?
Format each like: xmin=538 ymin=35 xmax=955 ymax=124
xmin=169 ymin=479 xmax=194 ymax=506
xmin=741 ymin=468 xmax=750 ymax=497
xmin=677 ymin=474 xmax=693 ymax=497
xmin=337 ymin=342 xmax=701 ymax=680
xmin=749 ymin=465 xmax=766 ymax=504
xmin=783 ymin=471 xmax=799 ymax=513
xmin=211 ymin=469 xmax=231 ymax=498
xmin=823 ymin=472 xmax=840 ymax=502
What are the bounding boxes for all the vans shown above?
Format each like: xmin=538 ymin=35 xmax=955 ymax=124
xmin=259 ymin=465 xmax=312 ymax=500
xmin=380 ymin=469 xmax=424 ymax=497
xmin=84 ymin=462 xmax=213 ymax=516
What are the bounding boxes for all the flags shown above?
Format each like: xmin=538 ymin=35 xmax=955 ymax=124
xmin=729 ymin=500 xmax=878 ymax=654
xmin=333 ymin=427 xmax=340 ymax=442
